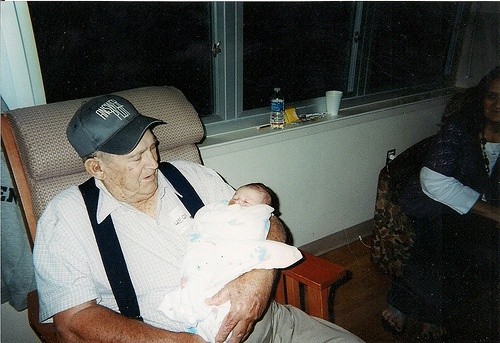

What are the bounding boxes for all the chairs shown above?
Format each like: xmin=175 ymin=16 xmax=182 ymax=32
xmin=0 ymin=85 xmax=346 ymax=343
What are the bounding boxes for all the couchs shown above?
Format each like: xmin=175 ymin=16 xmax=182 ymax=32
xmin=371 ymin=93 xmax=467 ymax=299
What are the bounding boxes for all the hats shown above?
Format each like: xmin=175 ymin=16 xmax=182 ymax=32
xmin=66 ymin=94 xmax=163 ymax=158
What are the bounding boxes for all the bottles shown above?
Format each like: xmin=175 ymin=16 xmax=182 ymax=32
xmin=270 ymin=88 xmax=284 ymax=129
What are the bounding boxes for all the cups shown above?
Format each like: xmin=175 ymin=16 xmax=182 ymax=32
xmin=326 ymin=91 xmax=343 ymax=115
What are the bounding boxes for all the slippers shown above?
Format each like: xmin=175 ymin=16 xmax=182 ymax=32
xmin=381 ymin=303 xmax=408 ymax=338
xmin=417 ymin=323 xmax=448 ymax=343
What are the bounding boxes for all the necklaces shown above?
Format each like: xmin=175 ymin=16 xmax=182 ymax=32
xmin=480 ymin=118 xmax=490 ymax=179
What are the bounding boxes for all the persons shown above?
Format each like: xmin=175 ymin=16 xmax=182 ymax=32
xmin=158 ymin=183 xmax=303 ymax=342
xmin=33 ymin=94 xmax=365 ymax=343
xmin=381 ymin=65 xmax=500 ymax=343
